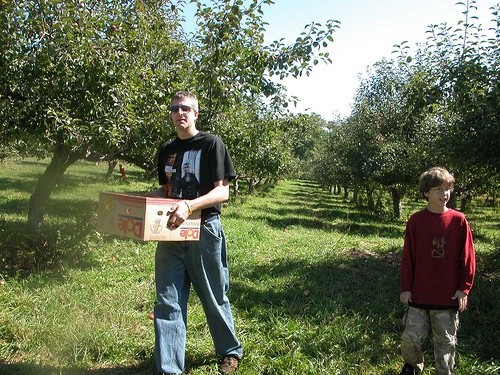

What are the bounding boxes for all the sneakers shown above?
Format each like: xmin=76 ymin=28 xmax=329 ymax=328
xmin=399 ymin=362 xmax=419 ymax=375
xmin=218 ymin=353 xmax=239 ymax=375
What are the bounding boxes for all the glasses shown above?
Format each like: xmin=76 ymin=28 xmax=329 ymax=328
xmin=169 ymin=106 xmax=197 ymax=113
xmin=425 ymin=187 xmax=454 ymax=193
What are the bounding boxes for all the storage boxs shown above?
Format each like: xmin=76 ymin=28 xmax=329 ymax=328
xmin=96 ymin=192 xmax=201 ymax=241
